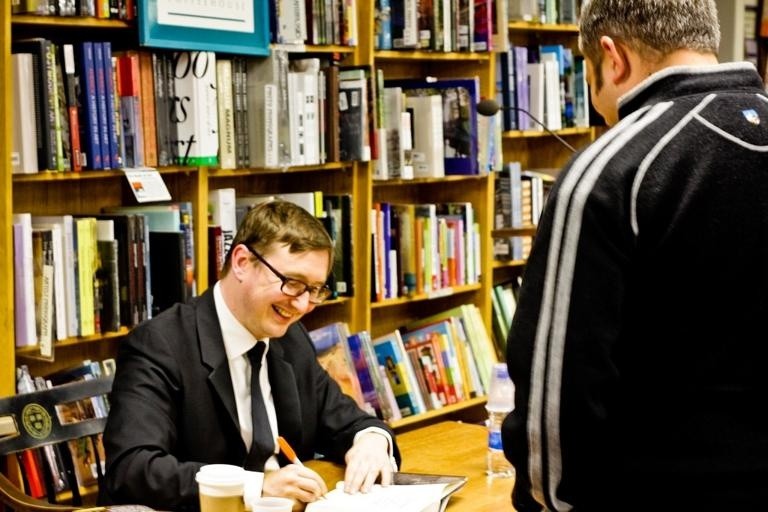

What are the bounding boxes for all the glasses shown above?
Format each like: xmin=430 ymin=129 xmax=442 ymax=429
xmin=242 ymin=241 xmax=332 ymax=304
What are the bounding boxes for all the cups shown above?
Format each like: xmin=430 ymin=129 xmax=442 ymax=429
xmin=196 ymin=463 xmax=294 ymax=512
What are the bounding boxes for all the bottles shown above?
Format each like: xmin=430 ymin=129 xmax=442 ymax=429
xmin=484 ymin=364 xmax=519 ymax=477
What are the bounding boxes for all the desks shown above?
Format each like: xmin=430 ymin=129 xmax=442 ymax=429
xmin=290 ymin=409 xmax=522 ymax=512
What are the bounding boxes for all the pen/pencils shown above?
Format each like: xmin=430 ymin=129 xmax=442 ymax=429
xmin=277 ymin=436 xmax=329 ymax=500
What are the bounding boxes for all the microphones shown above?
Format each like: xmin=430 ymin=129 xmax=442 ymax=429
xmin=475 ymin=99 xmax=578 ymax=156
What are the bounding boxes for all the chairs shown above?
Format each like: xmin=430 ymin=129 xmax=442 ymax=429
xmin=0 ymin=369 xmax=156 ymax=510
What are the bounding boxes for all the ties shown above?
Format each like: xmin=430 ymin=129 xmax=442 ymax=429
xmin=243 ymin=341 xmax=275 ymax=472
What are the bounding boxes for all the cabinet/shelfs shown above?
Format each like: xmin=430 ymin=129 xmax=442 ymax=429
xmin=711 ymin=0 xmax=767 ymax=85
xmin=0 ymin=2 xmax=618 ymax=511
